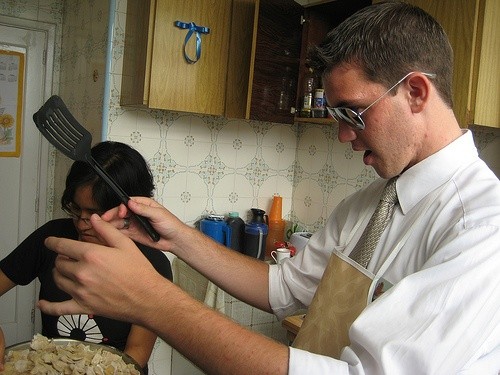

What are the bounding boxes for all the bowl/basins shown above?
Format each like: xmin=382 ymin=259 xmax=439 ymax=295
xmin=0 ymin=338 xmax=145 ymax=375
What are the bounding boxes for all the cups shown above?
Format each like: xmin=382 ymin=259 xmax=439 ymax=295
xmin=271 ymin=248 xmax=290 ymax=264
xmin=198 ymin=218 xmax=232 ymax=248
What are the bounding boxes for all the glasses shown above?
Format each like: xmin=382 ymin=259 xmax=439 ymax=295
xmin=326 ymin=70 xmax=435 ymax=132
xmin=61 ymin=205 xmax=91 ymax=227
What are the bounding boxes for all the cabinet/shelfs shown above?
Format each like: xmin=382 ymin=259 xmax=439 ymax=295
xmin=120 ymin=0 xmax=500 ymax=130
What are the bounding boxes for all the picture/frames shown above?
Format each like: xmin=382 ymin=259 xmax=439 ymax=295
xmin=0 ymin=50 xmax=24 ymax=157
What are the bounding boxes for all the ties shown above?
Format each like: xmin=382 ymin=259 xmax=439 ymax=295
xmin=346 ymin=175 xmax=398 ymax=271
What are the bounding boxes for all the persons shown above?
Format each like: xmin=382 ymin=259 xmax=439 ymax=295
xmin=0 ymin=141 xmax=173 ymax=375
xmin=37 ymin=0 xmax=500 ymax=375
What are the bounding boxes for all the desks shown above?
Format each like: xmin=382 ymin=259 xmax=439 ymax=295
xmin=173 ymin=258 xmax=289 ymax=375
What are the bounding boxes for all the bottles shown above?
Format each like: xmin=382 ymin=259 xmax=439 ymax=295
xmin=265 ymin=197 xmax=285 ymax=257
xmin=227 ymin=212 xmax=245 ymax=253
xmin=314 ymin=90 xmax=324 ymax=118
xmin=279 ymin=66 xmax=290 ymax=111
xmin=299 ymin=68 xmax=313 ymax=118
xmin=244 ymin=208 xmax=269 ymax=262
